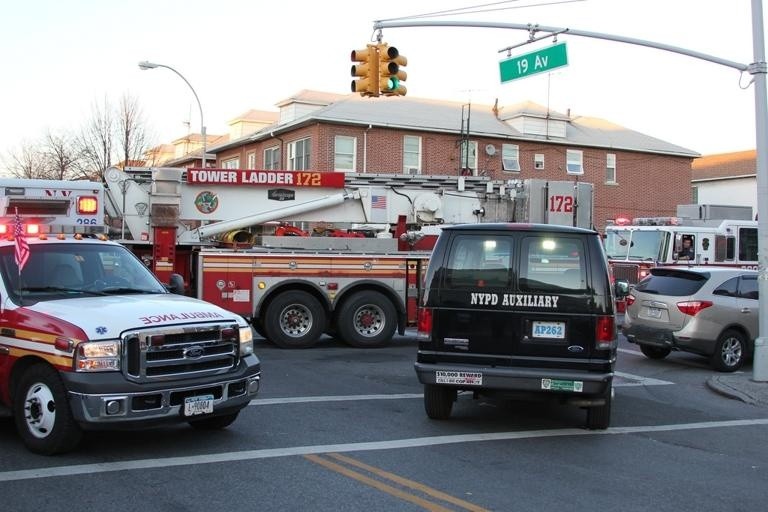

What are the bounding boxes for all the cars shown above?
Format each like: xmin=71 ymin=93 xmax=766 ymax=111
xmin=621 ymin=265 xmax=758 ymax=372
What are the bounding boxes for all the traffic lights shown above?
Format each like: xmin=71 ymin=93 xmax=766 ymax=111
xmin=350 ymin=43 xmax=407 ymax=97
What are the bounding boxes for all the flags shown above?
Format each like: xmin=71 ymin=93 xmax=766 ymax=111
xmin=370 ymin=195 xmax=386 ymax=210
xmin=14 ymin=209 xmax=30 ymax=276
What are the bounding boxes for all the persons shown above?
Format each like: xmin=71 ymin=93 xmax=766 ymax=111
xmin=678 ymin=237 xmax=694 ymax=260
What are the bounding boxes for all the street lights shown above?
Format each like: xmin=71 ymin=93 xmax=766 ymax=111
xmin=137 ymin=61 xmax=206 ymax=168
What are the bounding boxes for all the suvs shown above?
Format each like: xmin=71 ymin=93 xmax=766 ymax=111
xmin=415 ymin=222 xmax=630 ymax=430
xmin=0 ymin=233 xmax=262 ymax=456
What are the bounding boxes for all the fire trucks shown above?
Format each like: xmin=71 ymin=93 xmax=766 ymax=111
xmin=604 ymin=214 xmax=758 ymax=338
xmin=0 ymin=166 xmax=593 ymax=349
